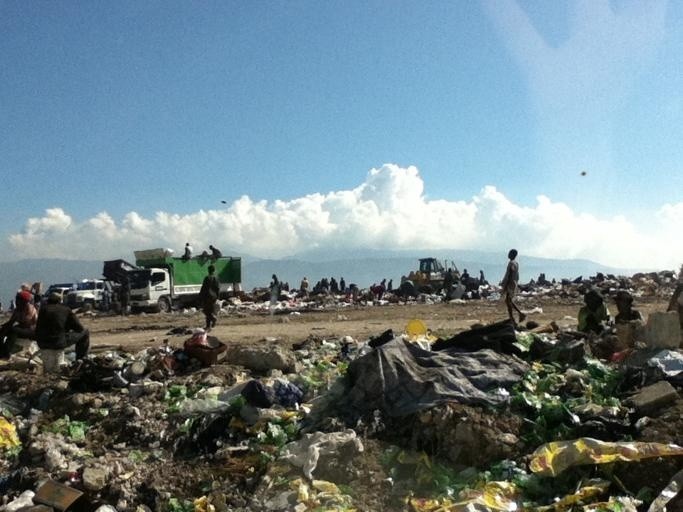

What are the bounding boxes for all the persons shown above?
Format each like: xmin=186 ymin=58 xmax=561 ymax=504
xmin=612 ymin=292 xmax=642 ymax=324
xmin=576 ymin=290 xmax=609 ymax=332
xmin=118 ymin=280 xmax=130 ymax=316
xmin=180 ymin=242 xmax=191 ymax=258
xmin=268 ymin=268 xmax=484 ymax=300
xmin=15 ymin=282 xmax=31 ymax=303
xmin=497 ymin=249 xmax=526 ymax=322
xmin=208 ymin=245 xmax=221 ymax=259
xmin=35 ymin=292 xmax=89 ymax=360
xmin=31 ymin=281 xmax=44 ymax=310
xmin=0 ymin=292 xmax=38 ymax=358
xmin=197 ymin=265 xmax=220 ymax=330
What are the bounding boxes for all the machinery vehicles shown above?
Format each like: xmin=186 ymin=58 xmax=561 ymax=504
xmin=400 ymin=255 xmax=461 ymax=297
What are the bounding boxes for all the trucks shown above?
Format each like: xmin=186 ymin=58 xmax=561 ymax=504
xmin=43 ymin=246 xmax=244 ymax=315
